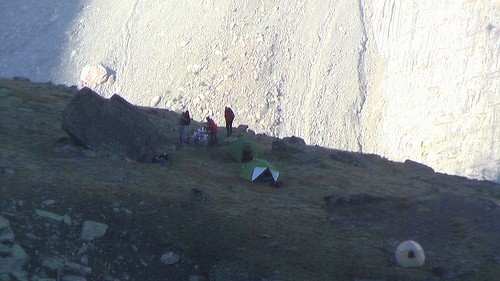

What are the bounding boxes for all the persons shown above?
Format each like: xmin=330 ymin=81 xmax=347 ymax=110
xmin=224 ymin=106 xmax=236 ymax=137
xmin=205 ymin=116 xmax=218 ymax=146
xmin=178 ymin=110 xmax=190 ymax=143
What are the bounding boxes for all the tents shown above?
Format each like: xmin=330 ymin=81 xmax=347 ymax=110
xmin=238 ymin=156 xmax=284 ymax=183
